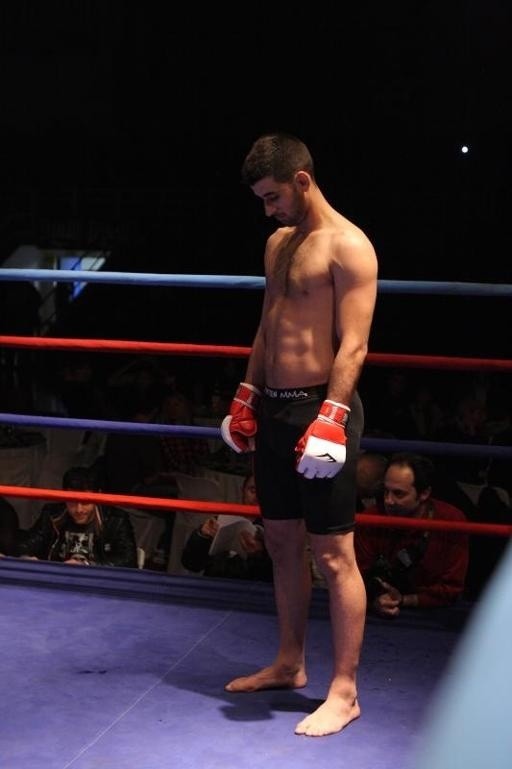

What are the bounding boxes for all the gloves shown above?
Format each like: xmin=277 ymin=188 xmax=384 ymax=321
xmin=293 ymin=399 xmax=352 ymax=481
xmin=221 ymin=382 xmax=262 ymax=455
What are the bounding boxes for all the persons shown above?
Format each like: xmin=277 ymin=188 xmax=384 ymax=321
xmin=219 ymin=133 xmax=381 ymax=742
xmin=2 ymin=340 xmax=511 ymax=619
